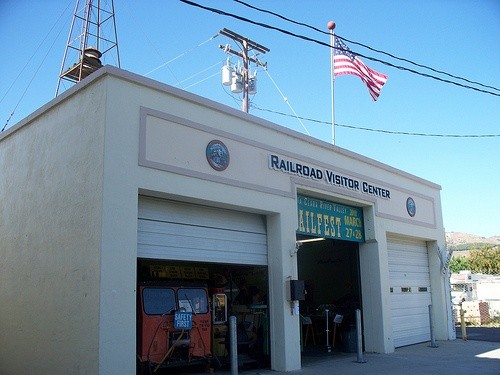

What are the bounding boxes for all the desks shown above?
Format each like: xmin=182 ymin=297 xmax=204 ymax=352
xmin=304 ymin=314 xmax=354 ymax=349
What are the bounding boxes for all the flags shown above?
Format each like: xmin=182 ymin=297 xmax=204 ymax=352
xmin=331 ymin=33 xmax=389 ymax=101
